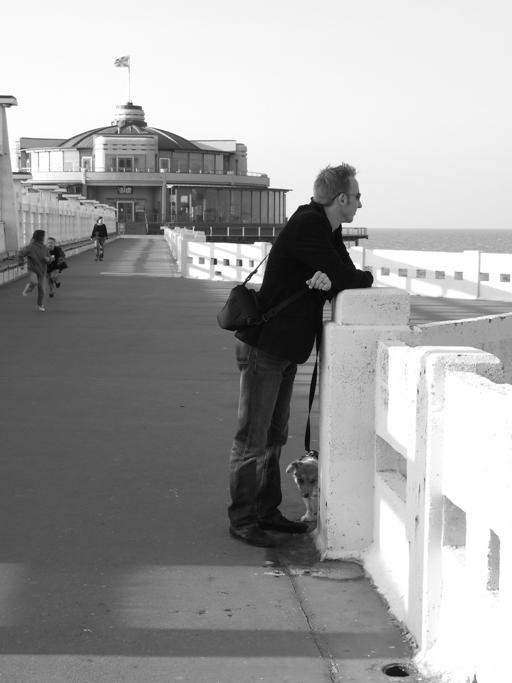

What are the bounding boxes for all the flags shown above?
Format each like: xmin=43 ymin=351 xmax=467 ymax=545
xmin=114 ymin=54 xmax=129 ymax=68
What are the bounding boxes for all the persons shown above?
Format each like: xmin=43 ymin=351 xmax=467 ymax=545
xmin=18 ymin=229 xmax=53 ymax=310
xmin=224 ymin=161 xmax=376 ymax=546
xmin=89 ymin=216 xmax=109 ymax=261
xmin=45 ymin=236 xmax=65 ymax=297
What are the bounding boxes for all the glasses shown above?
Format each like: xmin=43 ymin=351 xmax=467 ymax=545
xmin=346 ymin=190 xmax=362 ymax=201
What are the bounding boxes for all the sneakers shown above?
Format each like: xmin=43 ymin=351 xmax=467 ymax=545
xmin=23 ymin=282 xmax=60 ymax=311
xmin=258 ymin=508 xmax=308 ymax=533
xmin=229 ymin=521 xmax=276 ymax=547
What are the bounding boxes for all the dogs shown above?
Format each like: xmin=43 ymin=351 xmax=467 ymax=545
xmin=286 ymin=454 xmax=318 ymax=522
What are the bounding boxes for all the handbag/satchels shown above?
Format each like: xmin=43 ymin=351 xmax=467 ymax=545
xmin=216 ymin=283 xmax=263 ymax=332
